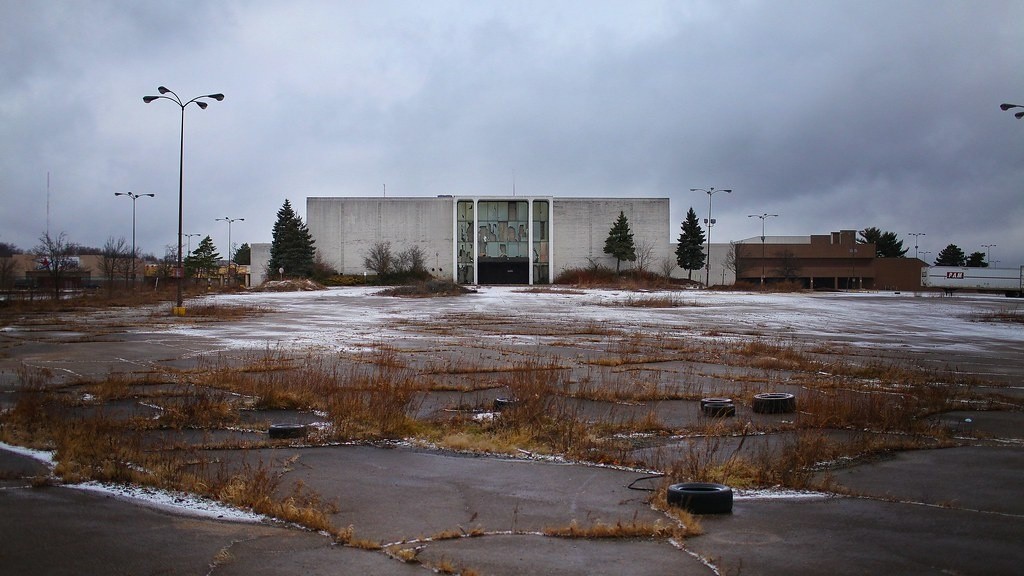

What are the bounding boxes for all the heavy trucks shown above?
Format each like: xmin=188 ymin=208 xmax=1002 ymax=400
xmin=921 ymin=265 xmax=1024 ymax=298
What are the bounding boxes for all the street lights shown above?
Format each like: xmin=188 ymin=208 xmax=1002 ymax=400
xmin=115 ymin=192 xmax=155 ymax=296
xmin=981 ymin=245 xmax=996 ymax=263
xmin=748 ymin=213 xmax=778 ymax=274
xmin=690 ymin=187 xmax=732 ymax=288
xmin=142 ymin=86 xmax=224 ymax=307
xmin=919 ymin=251 xmax=931 ymax=261
xmin=909 ymin=233 xmax=925 ymax=258
xmin=215 ymin=217 xmax=245 ymax=272
xmin=178 ymin=233 xmax=200 ymax=256
xmin=990 ymin=261 xmax=1001 ymax=267
